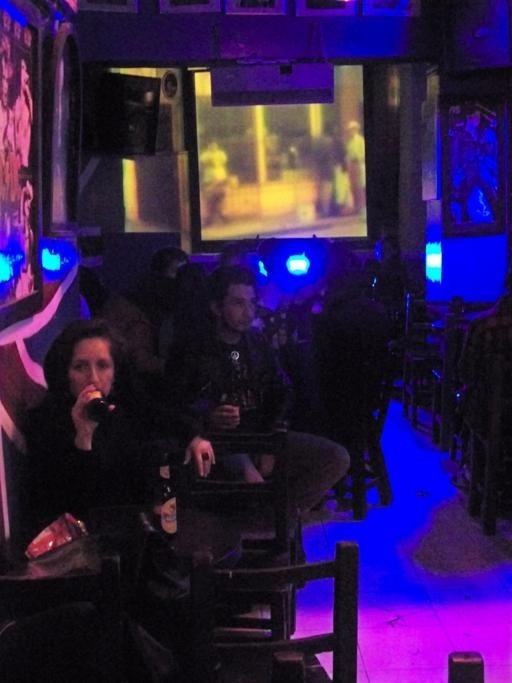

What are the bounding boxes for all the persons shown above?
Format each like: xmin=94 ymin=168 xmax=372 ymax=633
xmin=23 ymin=245 xmax=392 ymax=604
xmin=1 ymin=37 xmax=37 ymax=313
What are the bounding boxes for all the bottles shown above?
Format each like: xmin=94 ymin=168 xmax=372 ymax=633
xmin=86 ymin=386 xmax=108 ymax=422
xmin=220 ymin=381 xmax=239 ymax=408
xmin=159 ymin=453 xmax=177 ymax=540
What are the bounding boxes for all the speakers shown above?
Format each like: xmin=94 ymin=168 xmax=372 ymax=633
xmin=91 ymin=73 xmax=161 ymax=159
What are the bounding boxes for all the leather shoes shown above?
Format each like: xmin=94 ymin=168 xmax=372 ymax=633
xmin=276 ymin=507 xmax=300 ymax=547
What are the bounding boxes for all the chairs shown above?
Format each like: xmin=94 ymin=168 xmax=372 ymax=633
xmin=393 ymin=291 xmax=512 ymax=543
xmin=1 ymin=290 xmax=391 ymax=683
